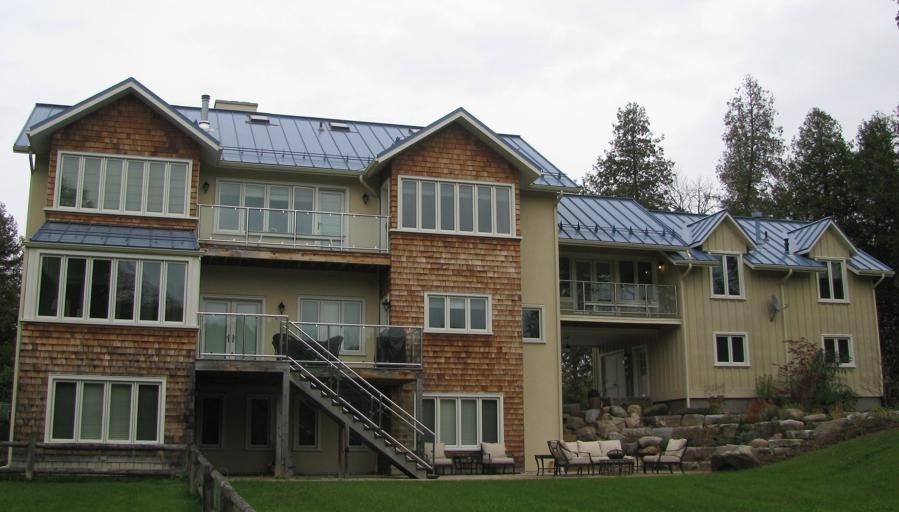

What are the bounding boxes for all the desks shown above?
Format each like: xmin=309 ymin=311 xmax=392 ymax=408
xmin=534 ymin=455 xmax=558 ymax=477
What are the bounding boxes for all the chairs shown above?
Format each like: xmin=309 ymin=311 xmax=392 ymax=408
xmin=547 ymin=439 xmax=595 ymax=477
xmin=642 ymin=437 xmax=689 ymax=474
xmin=423 ymin=442 xmax=516 ymax=475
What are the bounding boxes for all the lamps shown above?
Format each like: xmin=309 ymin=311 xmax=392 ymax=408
xmin=362 ymin=193 xmax=369 ymax=204
xmin=203 ymin=181 xmax=210 ymax=192
xmin=278 ymin=301 xmax=285 ymax=315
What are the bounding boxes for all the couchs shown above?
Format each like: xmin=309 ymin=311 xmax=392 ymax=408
xmin=559 ymin=439 xmax=635 ymax=476
